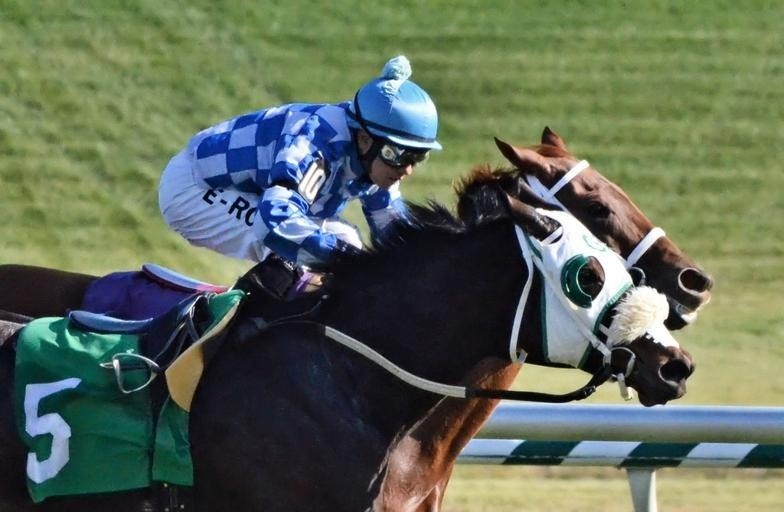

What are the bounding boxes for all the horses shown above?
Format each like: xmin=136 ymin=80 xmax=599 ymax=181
xmin=0 ymin=176 xmax=696 ymax=511
xmin=0 ymin=126 xmax=714 ymax=511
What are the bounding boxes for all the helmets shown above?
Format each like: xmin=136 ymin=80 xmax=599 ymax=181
xmin=346 ymin=77 xmax=443 ymax=150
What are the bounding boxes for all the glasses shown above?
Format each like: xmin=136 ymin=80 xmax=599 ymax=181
xmin=380 ymin=144 xmax=428 ymax=168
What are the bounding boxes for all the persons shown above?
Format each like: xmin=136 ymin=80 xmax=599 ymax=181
xmin=156 ymin=54 xmax=443 ymax=331
